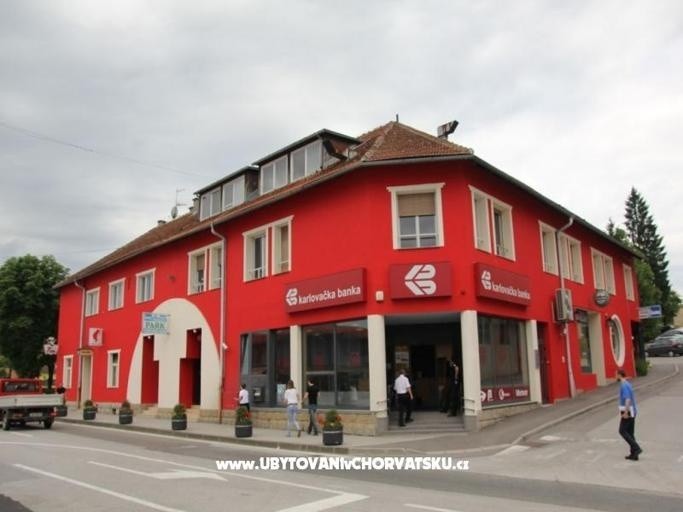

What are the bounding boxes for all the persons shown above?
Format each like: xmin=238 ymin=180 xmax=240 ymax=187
xmin=614 ymin=370 xmax=642 ymax=461
xmin=233 ymin=383 xmax=250 ymax=414
xmin=55 ymin=382 xmax=65 ymax=395
xmin=392 ymin=369 xmax=413 ymax=426
xmin=301 ymin=377 xmax=321 ymax=436
xmin=281 ymin=380 xmax=301 ymax=438
xmin=439 ymin=358 xmax=459 ymax=417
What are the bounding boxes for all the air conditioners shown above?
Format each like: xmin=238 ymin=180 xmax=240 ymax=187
xmin=554 ymin=285 xmax=575 ymax=324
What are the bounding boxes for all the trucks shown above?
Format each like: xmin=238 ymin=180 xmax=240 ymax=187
xmin=0 ymin=377 xmax=65 ymax=431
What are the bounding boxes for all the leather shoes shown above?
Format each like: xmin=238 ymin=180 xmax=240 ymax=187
xmin=625 ymin=448 xmax=642 ymax=460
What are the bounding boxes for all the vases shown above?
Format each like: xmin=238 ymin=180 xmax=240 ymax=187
xmin=118 ymin=414 xmax=132 ymax=424
xmin=171 ymin=419 xmax=187 ymax=430
xmin=83 ymin=410 xmax=95 ymax=419
xmin=235 ymin=424 xmax=252 ymax=437
xmin=322 ymin=431 xmax=342 ymax=444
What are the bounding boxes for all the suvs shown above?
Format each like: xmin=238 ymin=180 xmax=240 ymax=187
xmin=644 ymin=334 xmax=682 ymax=357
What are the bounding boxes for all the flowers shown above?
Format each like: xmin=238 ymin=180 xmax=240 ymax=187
xmin=118 ymin=399 xmax=132 ymax=413
xmin=170 ymin=404 xmax=186 ymax=419
xmin=233 ymin=404 xmax=251 ymax=425
xmin=82 ymin=399 xmax=97 ymax=411
xmin=317 ymin=409 xmax=343 ymax=431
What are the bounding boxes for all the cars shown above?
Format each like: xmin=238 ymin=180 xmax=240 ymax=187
xmin=655 ymin=326 xmax=682 ymax=340
